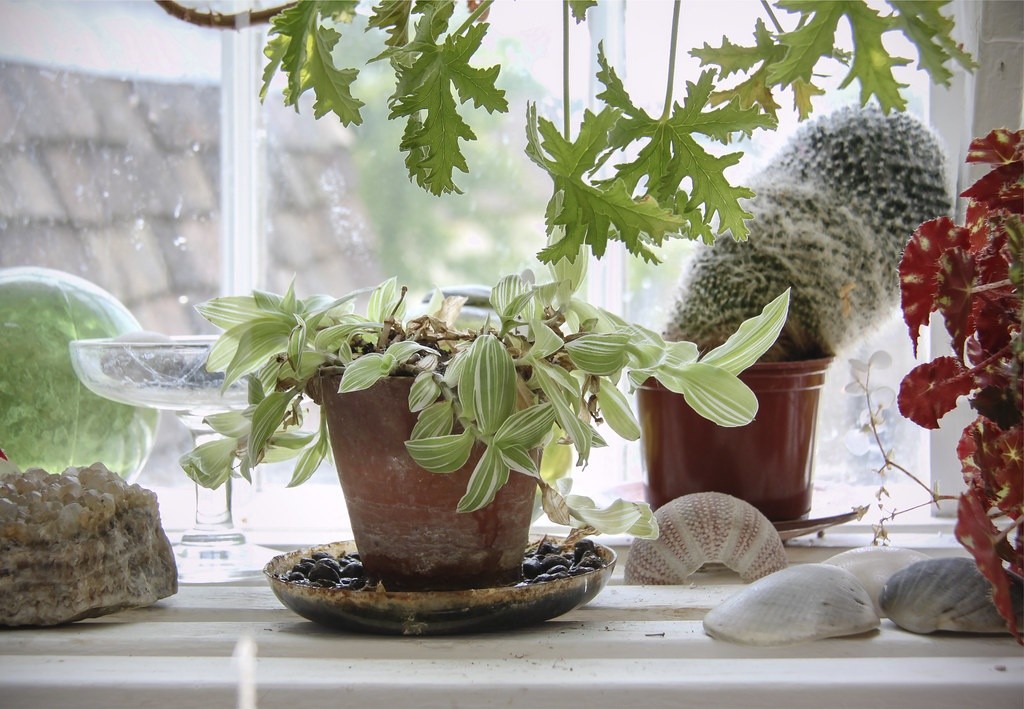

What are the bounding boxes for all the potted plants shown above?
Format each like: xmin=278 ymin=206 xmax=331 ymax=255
xmin=176 ymin=0 xmax=981 ymax=589
